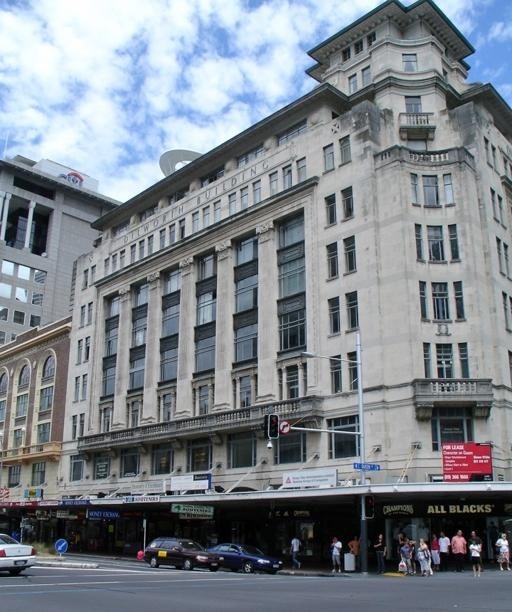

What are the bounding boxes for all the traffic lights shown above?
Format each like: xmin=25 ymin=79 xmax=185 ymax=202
xmin=268 ymin=414 xmax=280 ymax=440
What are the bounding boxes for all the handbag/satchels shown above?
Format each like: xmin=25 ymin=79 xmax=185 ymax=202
xmin=496 ymin=547 xmax=500 ymax=553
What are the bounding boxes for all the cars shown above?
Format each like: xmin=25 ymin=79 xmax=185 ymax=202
xmin=205 ymin=541 xmax=285 ymax=575
xmin=0 ymin=532 xmax=38 ymax=575
xmin=141 ymin=536 xmax=225 ymax=572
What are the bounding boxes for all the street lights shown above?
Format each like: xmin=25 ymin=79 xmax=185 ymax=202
xmin=299 ymin=349 xmax=371 ymax=574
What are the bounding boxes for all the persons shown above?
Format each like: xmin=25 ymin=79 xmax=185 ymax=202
xmin=396 ymin=527 xmax=483 ymax=577
xmin=347 ymin=535 xmax=362 ymax=570
xmin=289 ymin=534 xmax=302 ymax=569
xmin=329 ymin=536 xmax=343 ymax=573
xmin=373 ymin=532 xmax=387 ymax=574
xmin=493 ymin=530 xmax=511 ymax=571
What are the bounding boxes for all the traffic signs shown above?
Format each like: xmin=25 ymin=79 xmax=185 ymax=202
xmin=352 ymin=463 xmax=381 ymax=472
